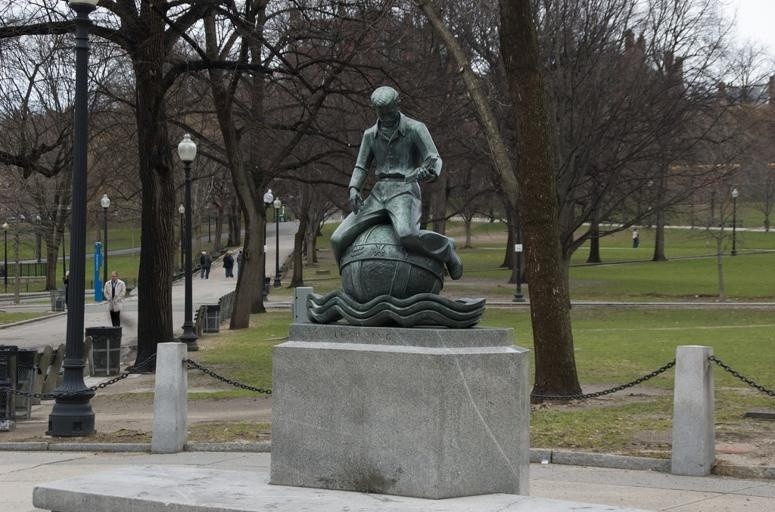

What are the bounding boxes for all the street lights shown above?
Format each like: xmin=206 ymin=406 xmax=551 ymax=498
xmin=731 ymin=188 xmax=740 ymax=257
xmin=177 ymin=133 xmax=200 ymax=353
xmin=262 ymin=188 xmax=282 ymax=301
xmin=2 ymin=222 xmax=10 ymax=294
xmin=99 ymin=193 xmax=111 ymax=301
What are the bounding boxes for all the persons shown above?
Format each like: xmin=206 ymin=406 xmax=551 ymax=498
xmin=633 ymin=229 xmax=639 ymax=247
xmin=104 ymin=271 xmax=126 ymax=326
xmin=64 ymin=271 xmax=70 ymax=309
xmin=200 ymin=251 xmax=243 ymax=279
xmin=330 ymin=85 xmax=463 ymax=280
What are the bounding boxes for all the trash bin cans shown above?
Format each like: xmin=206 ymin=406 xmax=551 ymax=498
xmin=201 ymin=305 xmax=220 ymax=333
xmin=86 ymin=326 xmax=122 ymax=377
